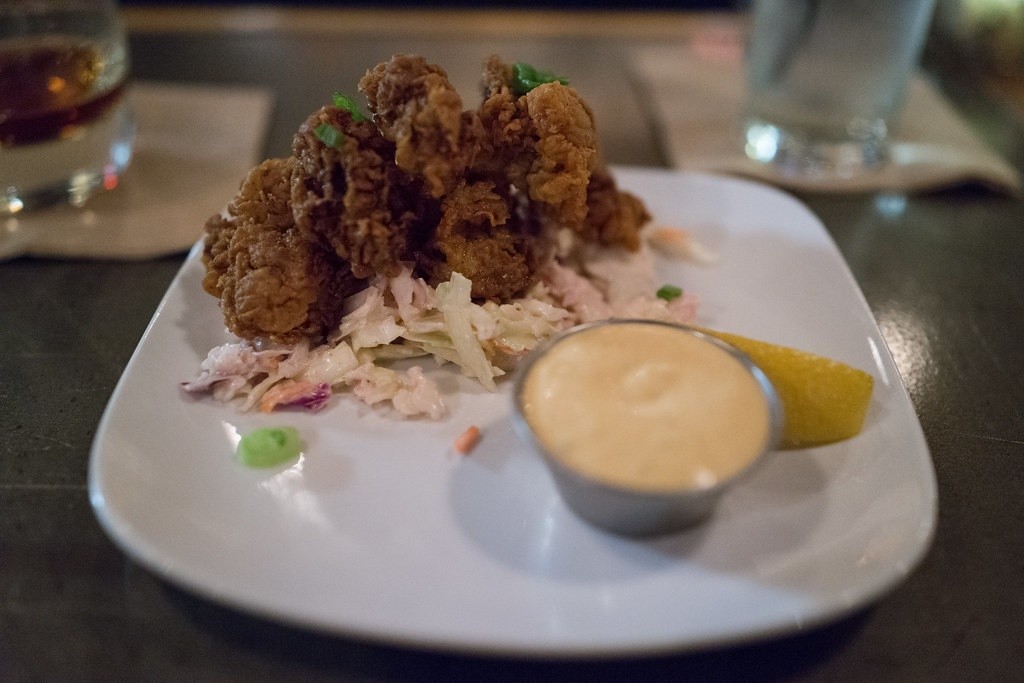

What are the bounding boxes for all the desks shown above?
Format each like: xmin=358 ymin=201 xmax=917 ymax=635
xmin=0 ymin=10 xmax=1024 ymax=683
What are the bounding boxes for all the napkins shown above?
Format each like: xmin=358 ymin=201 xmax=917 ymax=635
xmin=620 ymin=42 xmax=1024 ymax=200
xmin=0 ymin=81 xmax=276 ymax=259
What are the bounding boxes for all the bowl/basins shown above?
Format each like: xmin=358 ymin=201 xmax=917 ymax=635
xmin=515 ymin=318 xmax=784 ymax=532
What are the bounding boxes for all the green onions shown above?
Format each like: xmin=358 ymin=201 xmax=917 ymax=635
xmin=234 ymin=63 xmax=682 ymax=470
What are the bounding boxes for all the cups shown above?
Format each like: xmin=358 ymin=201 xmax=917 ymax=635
xmin=0 ymin=0 xmax=136 ymax=213
xmin=742 ymin=0 xmax=941 ymax=175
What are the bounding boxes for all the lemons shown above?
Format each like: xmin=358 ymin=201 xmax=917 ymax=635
xmin=699 ymin=329 xmax=875 ymax=451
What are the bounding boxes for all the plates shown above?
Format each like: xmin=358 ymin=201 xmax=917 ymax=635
xmin=89 ymin=163 xmax=938 ymax=657
